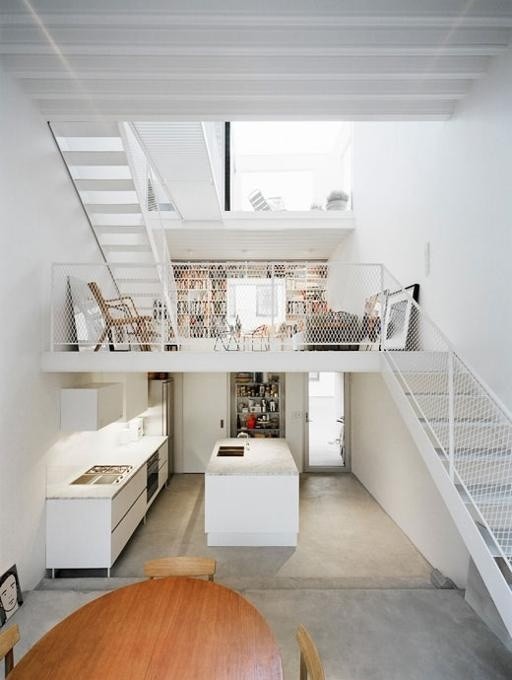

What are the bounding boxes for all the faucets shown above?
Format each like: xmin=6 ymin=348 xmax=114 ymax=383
xmin=237 ymin=432 xmax=250 ymax=451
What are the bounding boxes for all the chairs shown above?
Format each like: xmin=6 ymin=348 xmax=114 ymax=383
xmin=293 ymin=624 xmax=327 ymax=680
xmin=247 ymin=186 xmax=272 ymax=210
xmin=143 ymin=556 xmax=217 ymax=582
xmin=87 ymin=281 xmax=161 ymax=352
xmin=213 ymin=313 xmax=298 ymax=351
xmin=0 ymin=623 xmax=21 ymax=678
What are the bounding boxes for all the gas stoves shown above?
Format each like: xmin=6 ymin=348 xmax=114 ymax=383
xmin=69 ymin=465 xmax=132 ymax=486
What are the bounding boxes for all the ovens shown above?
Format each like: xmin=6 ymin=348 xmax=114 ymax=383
xmin=147 ymin=451 xmax=159 ymax=504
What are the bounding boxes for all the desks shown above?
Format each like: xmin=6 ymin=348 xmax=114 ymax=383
xmin=5 ymin=576 xmax=283 ymax=679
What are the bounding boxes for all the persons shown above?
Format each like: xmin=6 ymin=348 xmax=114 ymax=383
xmin=0 ymin=570 xmax=24 ymax=626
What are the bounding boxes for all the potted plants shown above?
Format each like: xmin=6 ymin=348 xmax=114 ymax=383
xmin=327 ymin=189 xmax=349 ymax=210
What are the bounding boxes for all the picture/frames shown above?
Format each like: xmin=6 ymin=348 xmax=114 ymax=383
xmin=382 ymin=284 xmax=420 ymax=351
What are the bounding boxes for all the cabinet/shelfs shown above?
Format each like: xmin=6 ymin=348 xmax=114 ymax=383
xmin=175 ymin=257 xmax=329 ymax=338
xmin=230 ymin=371 xmax=284 ymax=435
xmin=43 ymin=436 xmax=171 ymax=578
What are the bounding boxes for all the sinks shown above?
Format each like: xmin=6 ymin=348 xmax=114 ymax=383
xmin=217 ymin=450 xmax=244 ymax=456
xmin=219 ymin=446 xmax=244 ymax=451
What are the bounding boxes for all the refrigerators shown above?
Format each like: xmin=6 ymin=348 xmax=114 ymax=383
xmin=148 ymin=377 xmax=173 ymax=478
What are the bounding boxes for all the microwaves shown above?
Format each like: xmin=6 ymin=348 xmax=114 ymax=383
xmin=60 ymin=382 xmax=123 ymax=432
